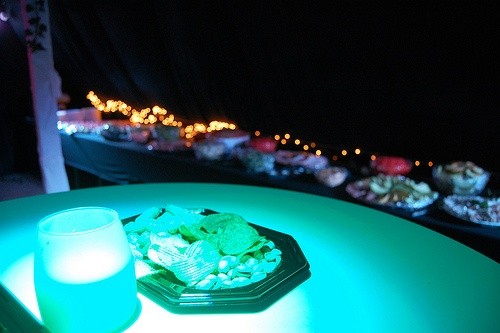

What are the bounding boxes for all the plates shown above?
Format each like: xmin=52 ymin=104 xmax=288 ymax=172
xmin=441 ymin=195 xmax=500 ymax=227
xmin=120 ymin=208 xmax=312 ymax=314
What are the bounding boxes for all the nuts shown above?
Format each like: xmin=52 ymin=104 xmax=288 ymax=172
xmin=124 ymin=204 xmax=282 ymax=290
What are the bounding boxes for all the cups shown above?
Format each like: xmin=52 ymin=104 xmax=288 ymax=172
xmin=34 ymin=206 xmax=142 ymax=332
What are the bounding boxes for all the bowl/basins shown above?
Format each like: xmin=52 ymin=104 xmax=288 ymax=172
xmin=432 ymin=169 xmax=490 ymax=196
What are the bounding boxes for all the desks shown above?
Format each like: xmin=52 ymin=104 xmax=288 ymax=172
xmin=0 ymin=183 xmax=500 ymax=332
xmin=57 ymin=108 xmax=500 ymax=243
